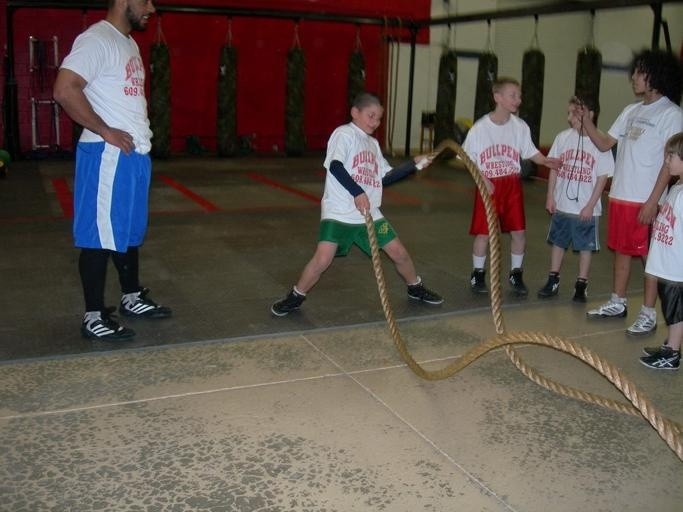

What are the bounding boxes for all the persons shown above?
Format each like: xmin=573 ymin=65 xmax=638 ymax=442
xmin=535 ymin=92 xmax=616 ymax=304
xmin=573 ymin=46 xmax=683 ymax=338
xmin=50 ymin=0 xmax=173 ymax=345
xmin=454 ymin=75 xmax=564 ymax=297
xmin=636 ymin=130 xmax=683 ymax=371
xmin=270 ymin=91 xmax=445 ymax=317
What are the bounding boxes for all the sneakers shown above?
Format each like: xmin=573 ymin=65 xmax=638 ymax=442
xmin=271 ymin=285 xmax=307 ymax=317
xmin=407 ymin=276 xmax=443 ymax=304
xmin=470 ymin=267 xmax=681 ymax=370
xmin=119 ymin=287 xmax=171 ymax=320
xmin=81 ymin=306 xmax=137 ymax=342
